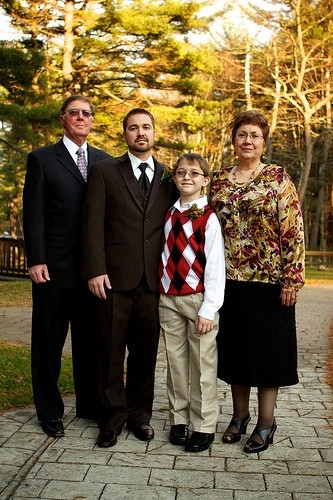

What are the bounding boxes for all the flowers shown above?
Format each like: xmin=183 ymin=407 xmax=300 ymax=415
xmin=184 ymin=203 xmax=205 ymax=219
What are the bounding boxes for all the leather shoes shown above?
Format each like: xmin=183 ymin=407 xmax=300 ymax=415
xmin=40 ymin=415 xmax=65 ymax=438
xmin=169 ymin=424 xmax=189 ymax=445
xmin=132 ymin=422 xmax=155 ymax=441
xmin=97 ymin=426 xmax=122 ymax=447
xmin=186 ymin=431 xmax=215 ymax=452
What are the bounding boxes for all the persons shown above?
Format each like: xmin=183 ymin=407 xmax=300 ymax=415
xmin=84 ymin=109 xmax=178 ymax=447
xmin=157 ymin=152 xmax=225 ymax=454
xmin=22 ymin=97 xmax=117 ymax=437
xmin=207 ymin=111 xmax=307 ymax=452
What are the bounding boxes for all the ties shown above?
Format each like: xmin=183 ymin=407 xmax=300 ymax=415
xmin=76 ymin=148 xmax=88 ymax=181
xmin=138 ymin=162 xmax=152 ymax=195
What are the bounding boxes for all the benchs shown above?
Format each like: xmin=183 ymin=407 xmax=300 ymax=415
xmin=305 ymin=251 xmax=333 ymax=265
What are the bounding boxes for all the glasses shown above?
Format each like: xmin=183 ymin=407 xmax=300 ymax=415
xmin=174 ymin=167 xmax=207 ymax=177
xmin=235 ymin=131 xmax=263 ymax=141
xmin=64 ymin=107 xmax=94 ymax=116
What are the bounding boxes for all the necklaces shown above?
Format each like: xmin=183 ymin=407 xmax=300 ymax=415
xmin=233 ymin=163 xmax=261 ymax=185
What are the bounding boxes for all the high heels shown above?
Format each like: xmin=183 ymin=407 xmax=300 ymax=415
xmin=222 ymin=411 xmax=251 ymax=443
xmin=244 ymin=418 xmax=277 ymax=453
